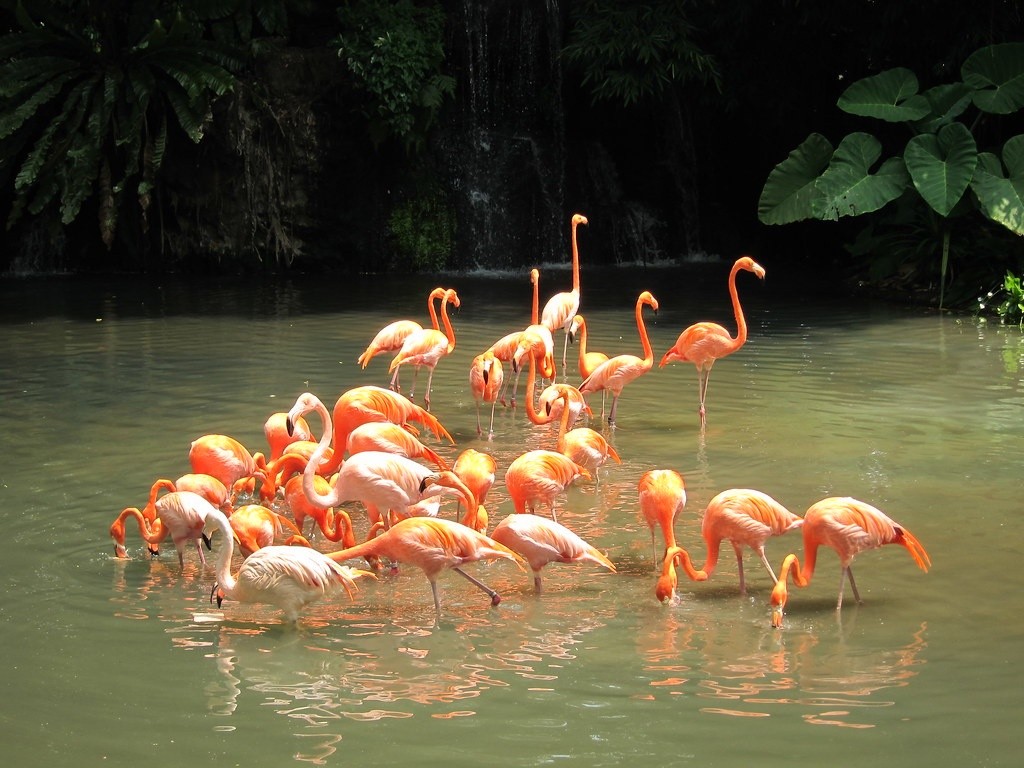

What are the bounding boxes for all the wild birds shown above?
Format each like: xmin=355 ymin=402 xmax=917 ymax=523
xmin=505 ymin=450 xmax=591 ymax=522
xmin=546 ymin=384 xmax=621 ymax=482
xmin=110 ymin=385 xmax=454 ymax=609
xmin=659 ymin=256 xmax=765 ymax=428
xmin=471 ymin=331 xmax=557 ymax=410
xmin=469 ymin=351 xmax=504 ymax=440
xmin=418 ymin=472 xmax=619 ymax=593
xmin=577 ymin=291 xmax=659 ymax=429
xmin=770 ymin=496 xmax=931 ymax=630
xmin=284 ymin=516 xmax=527 ymax=611
xmin=452 ymin=447 xmax=497 ymax=536
xmin=524 ymin=269 xmax=556 ymax=384
xmin=570 ymin=315 xmax=609 ymax=417
xmin=656 ymin=488 xmax=803 ymax=608
xmin=635 ymin=468 xmax=686 ymax=567
xmin=357 ymin=287 xmax=446 ymax=394
xmin=512 ymin=339 xmax=592 ymax=431
xmin=201 ymin=508 xmax=378 ymax=615
xmin=541 ymin=214 xmax=589 ymax=366
xmin=388 ymin=289 xmax=461 ymax=411
